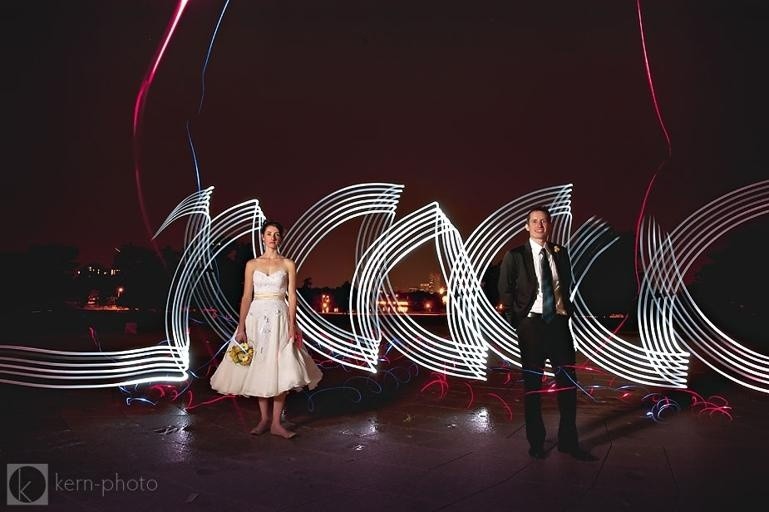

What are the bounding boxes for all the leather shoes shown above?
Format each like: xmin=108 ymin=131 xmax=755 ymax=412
xmin=557 ymin=445 xmax=595 ymax=462
xmin=528 ymin=444 xmax=545 ymax=459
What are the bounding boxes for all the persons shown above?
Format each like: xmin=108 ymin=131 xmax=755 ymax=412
xmin=496 ymin=207 xmax=596 ymax=464
xmin=210 ymin=219 xmax=326 ymax=441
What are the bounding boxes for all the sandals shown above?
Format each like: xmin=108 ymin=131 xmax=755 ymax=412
xmin=250 ymin=422 xmax=296 ymax=439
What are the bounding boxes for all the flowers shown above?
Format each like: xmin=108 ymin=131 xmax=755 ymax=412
xmin=228 ymin=341 xmax=253 ymax=367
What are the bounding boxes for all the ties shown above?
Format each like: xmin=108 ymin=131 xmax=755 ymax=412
xmin=539 ymin=248 xmax=556 ymax=323
